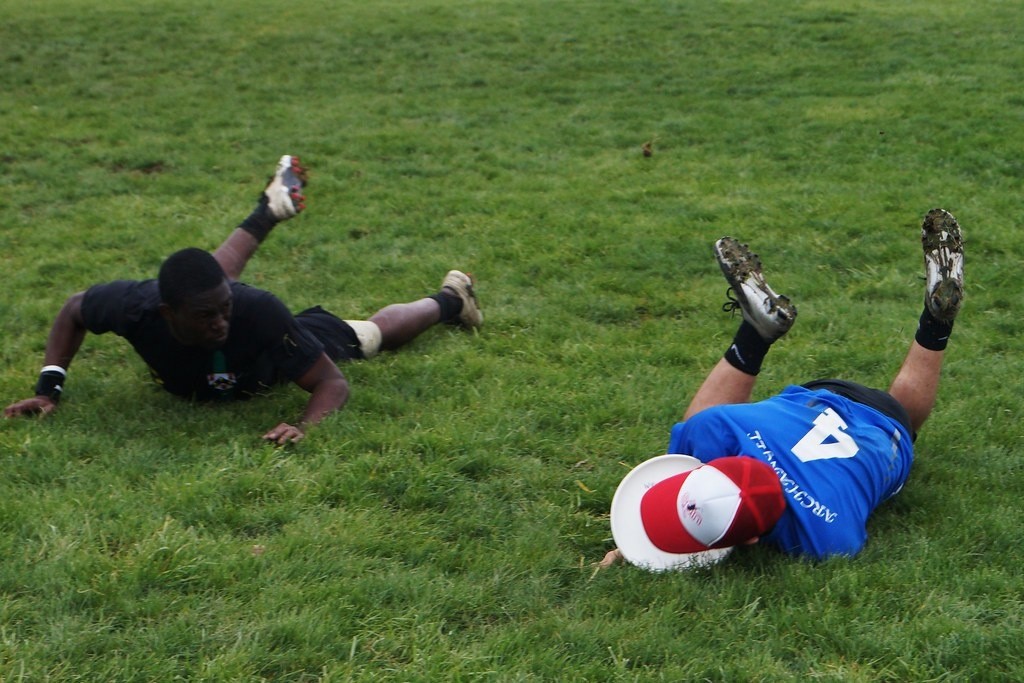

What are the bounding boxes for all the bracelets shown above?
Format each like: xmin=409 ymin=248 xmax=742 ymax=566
xmin=33 ymin=365 xmax=66 ymax=406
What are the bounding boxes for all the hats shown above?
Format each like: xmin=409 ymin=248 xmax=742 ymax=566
xmin=640 ymin=456 xmax=783 ymax=555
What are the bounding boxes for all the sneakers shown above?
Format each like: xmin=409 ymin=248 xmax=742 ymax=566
xmin=265 ymin=154 xmax=304 ymax=219
xmin=714 ymin=236 xmax=796 ymax=338
xmin=443 ymin=270 xmax=482 ymax=329
xmin=922 ymin=208 xmax=964 ymax=319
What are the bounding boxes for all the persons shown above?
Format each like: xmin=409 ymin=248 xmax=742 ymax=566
xmin=7 ymin=156 xmax=483 ymax=444
xmin=592 ymin=207 xmax=963 ymax=575
